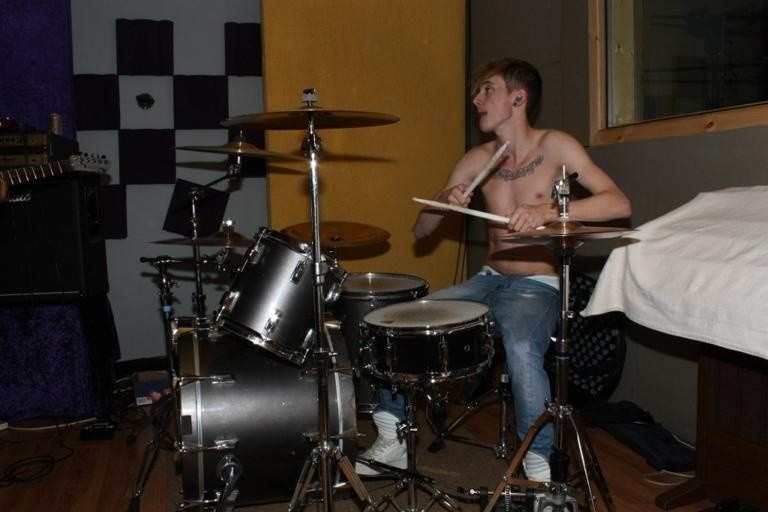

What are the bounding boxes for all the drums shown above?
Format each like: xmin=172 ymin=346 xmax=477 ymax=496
xmin=212 ymin=228 xmax=346 ymax=365
xmin=170 ymin=316 xmax=359 ymax=506
xmin=340 ymin=270 xmax=429 ymax=367
xmin=359 ymin=298 xmax=496 ymax=382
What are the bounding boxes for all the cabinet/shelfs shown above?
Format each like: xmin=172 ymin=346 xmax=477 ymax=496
xmin=619 ymin=183 xmax=767 ymax=512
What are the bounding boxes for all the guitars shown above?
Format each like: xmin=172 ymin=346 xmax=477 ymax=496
xmin=0 ymin=151 xmax=110 ymax=203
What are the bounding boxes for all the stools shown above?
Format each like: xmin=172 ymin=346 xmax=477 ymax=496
xmin=425 ymin=339 xmax=515 ymax=465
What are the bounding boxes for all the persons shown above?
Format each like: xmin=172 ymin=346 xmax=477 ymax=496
xmin=353 ymin=56 xmax=631 ymax=512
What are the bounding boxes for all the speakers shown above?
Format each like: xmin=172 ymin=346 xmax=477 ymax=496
xmin=0 ymin=179 xmax=109 ymax=306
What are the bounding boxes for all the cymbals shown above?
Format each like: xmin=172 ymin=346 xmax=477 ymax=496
xmin=152 ymin=231 xmax=256 ymax=248
xmin=220 ymin=108 xmax=400 ymax=128
xmin=172 ymin=144 xmax=305 ymax=163
xmin=493 ymin=223 xmax=641 ymax=239
xmin=284 ymin=220 xmax=389 ymax=247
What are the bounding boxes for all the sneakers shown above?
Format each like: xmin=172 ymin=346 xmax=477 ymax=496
xmin=532 ymin=491 xmax=570 ymax=512
xmin=355 ymin=436 xmax=408 ymax=475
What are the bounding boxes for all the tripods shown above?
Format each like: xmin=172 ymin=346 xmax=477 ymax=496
xmin=483 ymin=237 xmax=616 ymax=512
xmin=285 ymin=124 xmax=372 ymax=512
xmin=126 ymin=282 xmax=182 ymax=512
xmin=363 ymin=388 xmax=462 ymax=512
